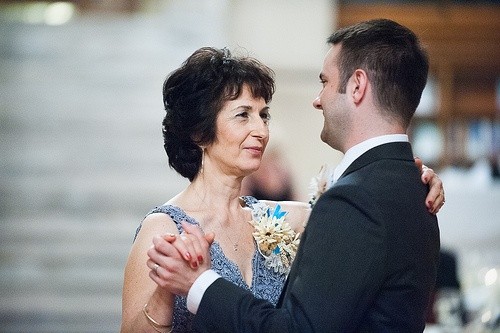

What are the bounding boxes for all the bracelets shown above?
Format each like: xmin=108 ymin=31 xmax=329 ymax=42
xmin=143 ymin=303 xmax=176 ymax=333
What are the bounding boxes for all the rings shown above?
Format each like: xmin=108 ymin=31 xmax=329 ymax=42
xmin=443 ymin=202 xmax=445 ymax=204
xmin=153 ymin=263 xmax=159 ymax=273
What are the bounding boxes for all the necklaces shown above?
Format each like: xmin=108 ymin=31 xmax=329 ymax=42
xmin=194 ymin=191 xmax=243 ymax=252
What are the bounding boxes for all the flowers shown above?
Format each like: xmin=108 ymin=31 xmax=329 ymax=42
xmin=250 ymin=205 xmax=305 ymax=281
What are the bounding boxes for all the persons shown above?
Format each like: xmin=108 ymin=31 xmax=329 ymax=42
xmin=147 ymin=19 xmax=440 ymax=333
xmin=119 ymin=44 xmax=446 ymax=333
